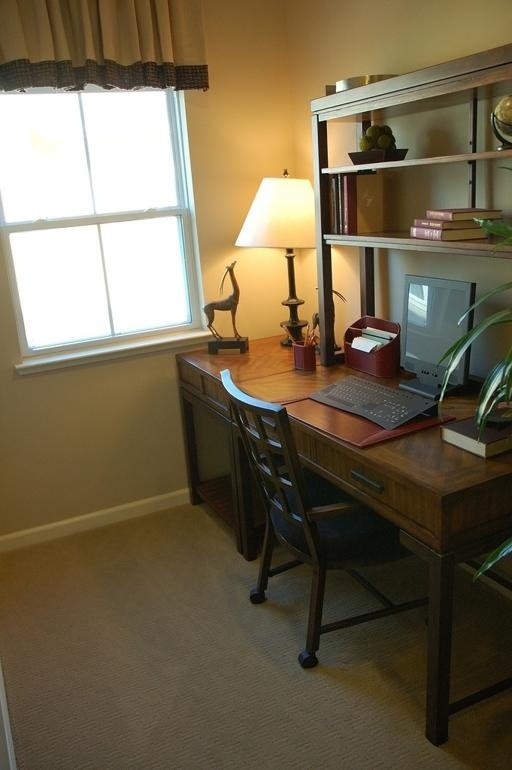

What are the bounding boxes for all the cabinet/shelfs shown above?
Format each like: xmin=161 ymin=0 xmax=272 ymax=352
xmin=309 ymin=42 xmax=510 ymax=369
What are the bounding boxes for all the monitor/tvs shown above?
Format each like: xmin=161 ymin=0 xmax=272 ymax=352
xmin=398 ymin=274 xmax=476 ymax=401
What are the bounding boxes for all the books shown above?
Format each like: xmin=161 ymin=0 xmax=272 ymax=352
xmin=410 ymin=208 xmax=503 ymax=241
xmin=439 ymin=408 xmax=512 ymax=460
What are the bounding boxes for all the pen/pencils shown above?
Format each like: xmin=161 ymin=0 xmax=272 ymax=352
xmin=284 ymin=324 xmax=316 ymax=346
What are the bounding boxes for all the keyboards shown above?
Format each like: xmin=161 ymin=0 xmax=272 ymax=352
xmin=309 ymin=375 xmax=438 ymax=431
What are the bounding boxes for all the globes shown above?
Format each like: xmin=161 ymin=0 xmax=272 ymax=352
xmin=491 ymin=94 xmax=512 ymax=152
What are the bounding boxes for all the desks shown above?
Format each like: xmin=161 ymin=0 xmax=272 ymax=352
xmin=175 ymin=334 xmax=512 ymax=747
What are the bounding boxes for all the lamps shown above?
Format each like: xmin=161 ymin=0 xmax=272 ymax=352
xmin=234 ymin=169 xmax=318 ymax=348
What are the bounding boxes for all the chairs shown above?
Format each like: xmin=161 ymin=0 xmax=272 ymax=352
xmin=214 ymin=367 xmax=431 ymax=669
